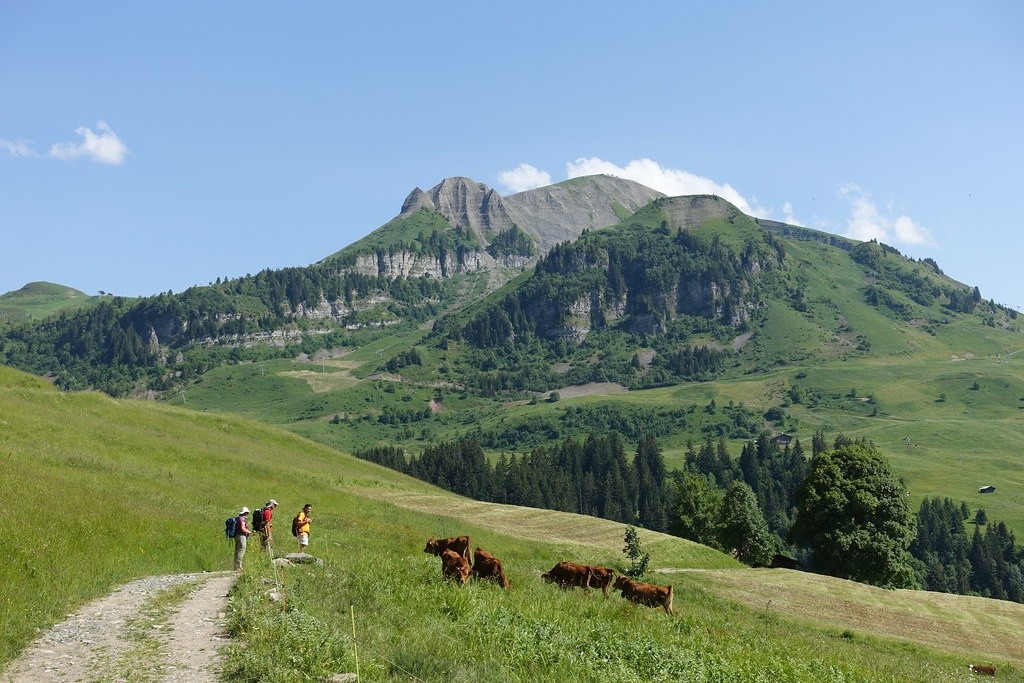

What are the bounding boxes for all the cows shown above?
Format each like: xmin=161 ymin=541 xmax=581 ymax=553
xmin=442 ymin=548 xmax=475 ymax=588
xmin=967 ymin=664 xmax=997 ymax=678
xmin=585 ymin=565 xmax=618 ymax=599
xmin=472 ymin=546 xmax=512 ymax=591
xmin=612 ymin=576 xmax=673 ymax=615
xmin=541 ymin=561 xmax=601 ymax=598
xmin=424 ymin=535 xmax=473 ymax=565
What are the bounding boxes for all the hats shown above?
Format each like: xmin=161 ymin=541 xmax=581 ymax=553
xmin=266 ymin=499 xmax=279 ymax=509
xmin=238 ymin=506 xmax=250 ymax=515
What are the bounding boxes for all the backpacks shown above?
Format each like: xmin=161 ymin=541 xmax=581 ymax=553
xmin=252 ymin=508 xmax=270 ymax=531
xmin=292 ymin=512 xmax=306 ymax=537
xmin=225 ymin=516 xmax=247 ymax=538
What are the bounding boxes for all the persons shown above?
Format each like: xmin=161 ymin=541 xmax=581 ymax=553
xmin=297 ymin=504 xmax=312 ymax=553
xmin=234 ymin=507 xmax=256 ymax=571
xmin=259 ymin=498 xmax=277 ymax=559
